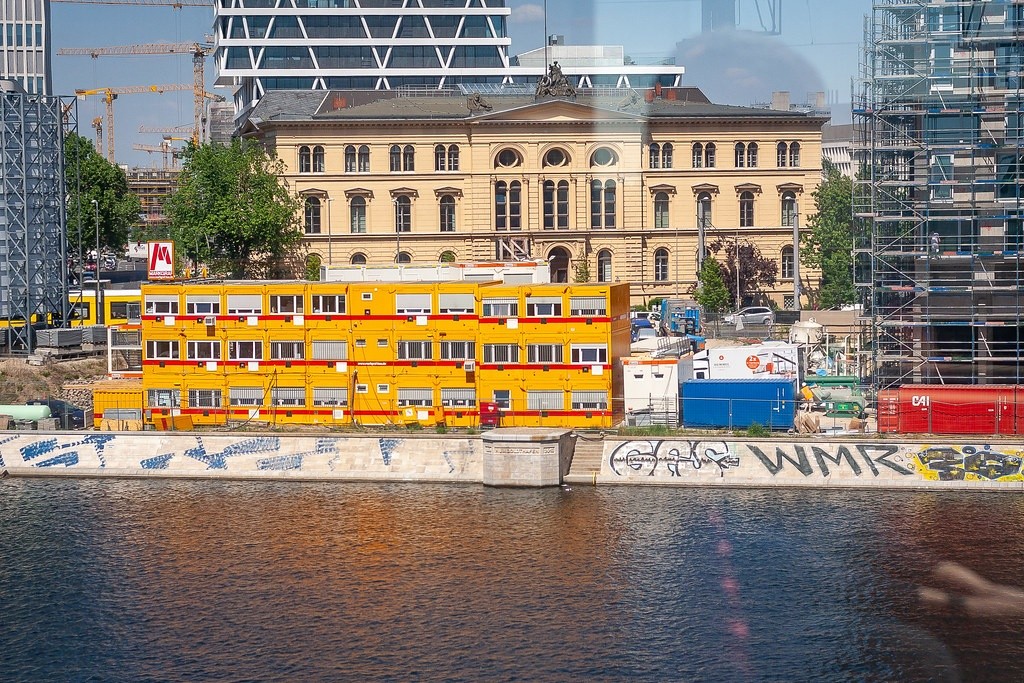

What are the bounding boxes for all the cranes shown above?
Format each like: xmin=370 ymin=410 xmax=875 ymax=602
xmin=51 ymin=0 xmax=228 ymax=210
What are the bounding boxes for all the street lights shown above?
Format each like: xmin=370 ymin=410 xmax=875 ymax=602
xmin=786 ymin=196 xmax=801 ymax=310
xmin=91 ymin=199 xmax=101 ymax=323
xmin=327 ymin=198 xmax=336 ymax=266
xmin=698 ymin=196 xmax=710 ymax=292
xmin=392 ymin=199 xmax=400 ymax=264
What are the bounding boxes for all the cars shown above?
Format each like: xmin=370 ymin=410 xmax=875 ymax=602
xmin=631 ymin=311 xmax=663 ymax=343
xmin=69 ymin=245 xmax=147 ymax=273
xmin=720 ymin=305 xmax=775 ymax=324
xmin=25 ymin=398 xmax=85 ymax=429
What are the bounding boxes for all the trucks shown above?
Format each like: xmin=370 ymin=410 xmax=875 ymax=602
xmin=693 ymin=342 xmax=800 ymax=379
